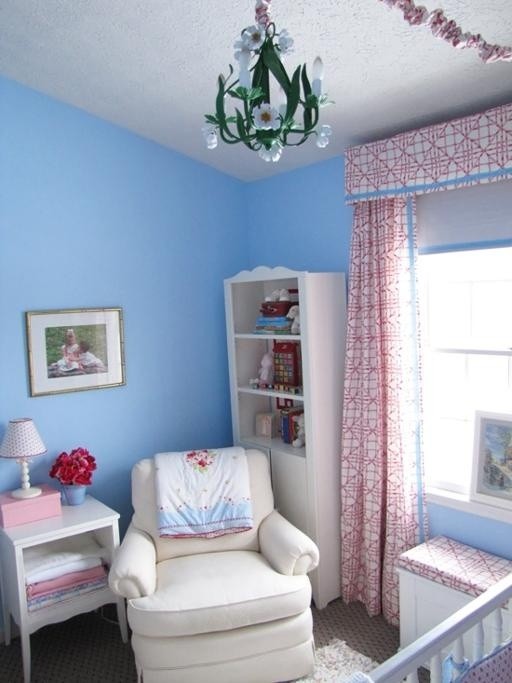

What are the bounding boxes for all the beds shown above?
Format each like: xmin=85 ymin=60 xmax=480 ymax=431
xmin=345 ymin=574 xmax=512 ymax=683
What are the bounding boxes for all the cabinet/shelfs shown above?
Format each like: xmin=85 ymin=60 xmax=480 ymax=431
xmin=0 ymin=494 xmax=130 ymax=683
xmin=223 ymin=265 xmax=347 ymax=610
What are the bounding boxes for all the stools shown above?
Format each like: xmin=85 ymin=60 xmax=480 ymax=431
xmin=394 ymin=534 xmax=512 ymax=683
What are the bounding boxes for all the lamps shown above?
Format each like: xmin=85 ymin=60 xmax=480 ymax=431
xmin=0 ymin=418 xmax=47 ymax=500
xmin=200 ymin=0 xmax=336 ymax=163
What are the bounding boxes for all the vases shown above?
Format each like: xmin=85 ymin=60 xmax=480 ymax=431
xmin=62 ymin=484 xmax=87 ymax=506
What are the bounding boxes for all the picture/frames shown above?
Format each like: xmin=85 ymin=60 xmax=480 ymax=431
xmin=25 ymin=307 xmax=127 ymax=398
xmin=468 ymin=409 xmax=512 ymax=511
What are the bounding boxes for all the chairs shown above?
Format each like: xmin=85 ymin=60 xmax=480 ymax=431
xmin=107 ymin=447 xmax=320 ymax=683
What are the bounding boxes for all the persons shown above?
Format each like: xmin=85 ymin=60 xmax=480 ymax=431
xmin=55 ymin=327 xmax=79 ymax=371
xmin=76 ymin=339 xmax=105 ymax=372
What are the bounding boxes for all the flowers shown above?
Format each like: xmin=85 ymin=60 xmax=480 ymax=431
xmin=49 ymin=447 xmax=97 ymax=486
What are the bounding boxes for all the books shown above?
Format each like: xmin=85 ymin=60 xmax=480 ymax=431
xmin=255 ymin=404 xmax=303 ymax=443
xmin=251 ymin=316 xmax=292 ymax=334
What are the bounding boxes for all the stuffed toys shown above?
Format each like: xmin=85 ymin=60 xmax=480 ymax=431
xmin=249 ymin=352 xmax=274 ymax=384
